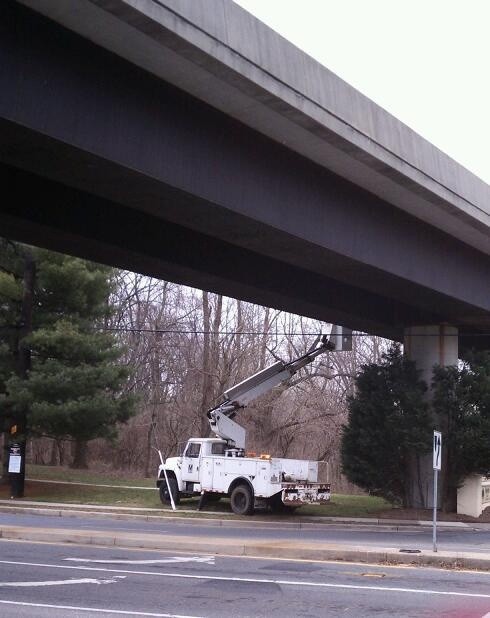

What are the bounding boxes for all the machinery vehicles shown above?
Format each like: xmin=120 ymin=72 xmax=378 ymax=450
xmin=155 ymin=322 xmax=353 ymax=515
